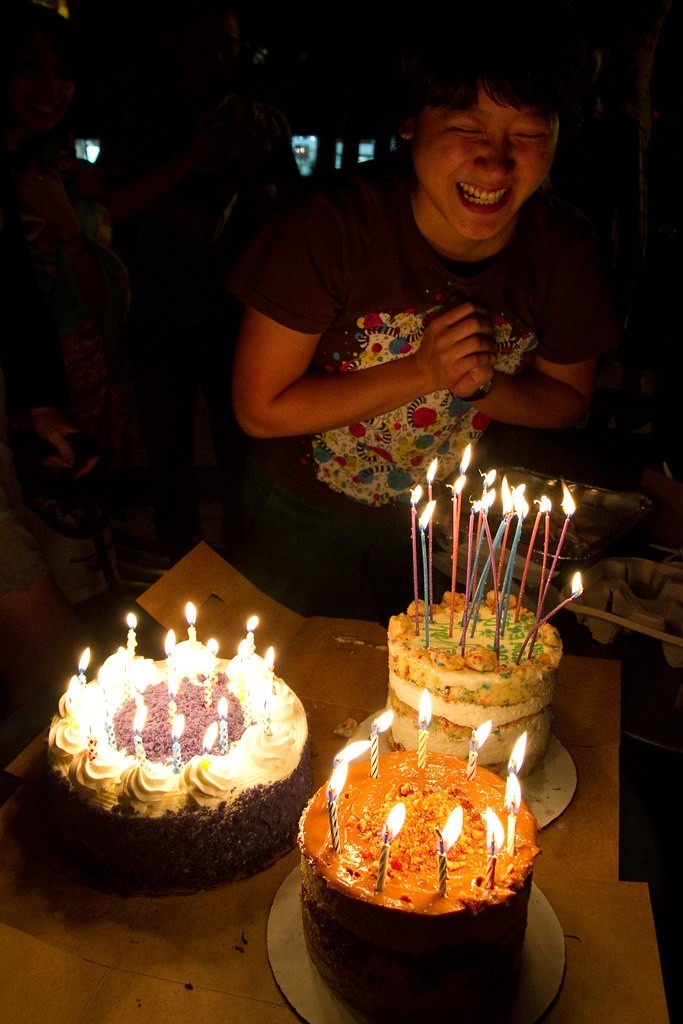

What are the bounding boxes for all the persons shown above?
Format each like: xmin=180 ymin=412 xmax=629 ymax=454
xmin=220 ymin=0 xmax=623 ymax=620
xmin=108 ymin=0 xmax=305 ymax=576
xmin=0 ymin=0 xmax=133 ymax=602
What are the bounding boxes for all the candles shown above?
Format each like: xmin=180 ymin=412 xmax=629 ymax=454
xmin=467 ymin=731 xmax=478 ymax=780
xmin=434 ymin=827 xmax=447 ymax=897
xmin=418 ymin=718 xmax=428 ymax=768
xmin=370 ymin=720 xmax=379 ymax=778
xmin=376 ymin=826 xmax=390 ymax=892
xmin=486 ymin=834 xmax=498 ymax=890
xmin=410 ymin=445 xmax=587 ymax=665
xmin=70 ymin=601 xmax=278 ymax=774
xmin=505 ymin=769 xmax=517 ymax=856
xmin=328 ymin=758 xmax=342 ymax=850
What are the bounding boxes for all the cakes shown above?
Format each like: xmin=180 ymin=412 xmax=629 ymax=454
xmin=383 ymin=589 xmax=564 ymax=781
xmin=46 ymin=641 xmax=310 ymax=894
xmin=297 ymin=749 xmax=542 ymax=1024
